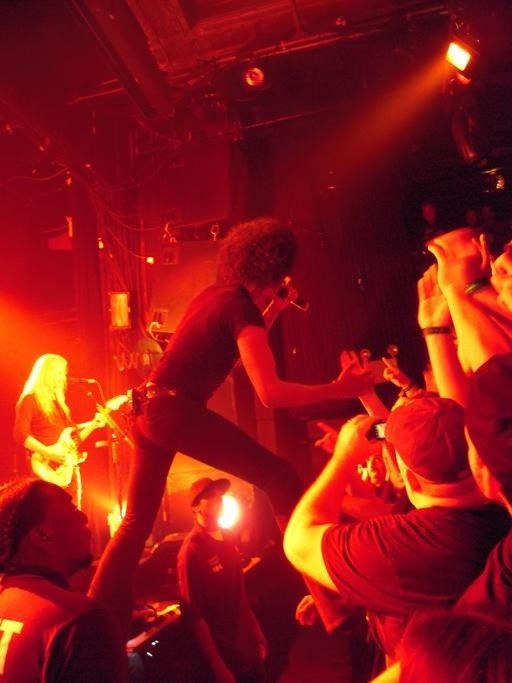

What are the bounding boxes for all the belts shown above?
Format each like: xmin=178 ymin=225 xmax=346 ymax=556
xmin=146 ymin=388 xmax=190 ymax=400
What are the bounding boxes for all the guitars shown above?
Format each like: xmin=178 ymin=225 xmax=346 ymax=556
xmin=31 ymin=395 xmax=128 ymax=489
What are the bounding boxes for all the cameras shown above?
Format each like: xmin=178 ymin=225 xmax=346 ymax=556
xmin=368 ymin=422 xmax=387 ymax=439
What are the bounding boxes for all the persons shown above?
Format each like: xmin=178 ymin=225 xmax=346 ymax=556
xmin=1 ymin=476 xmax=134 ymax=682
xmin=278 ymin=200 xmax=511 ymax=682
xmin=12 ymin=352 xmax=108 ymax=512
xmin=87 ymin=214 xmax=378 ymax=642
xmin=174 ymin=474 xmax=275 ymax=682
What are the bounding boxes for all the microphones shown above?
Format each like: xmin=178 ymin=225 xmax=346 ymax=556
xmin=278 ymin=288 xmax=310 ymax=311
xmin=67 ymin=378 xmax=95 ymax=383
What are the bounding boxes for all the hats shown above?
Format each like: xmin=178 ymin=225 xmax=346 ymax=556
xmin=389 ymin=398 xmax=463 ymax=480
xmin=464 ymin=350 xmax=512 ymax=498
xmin=189 ymin=478 xmax=230 ymax=506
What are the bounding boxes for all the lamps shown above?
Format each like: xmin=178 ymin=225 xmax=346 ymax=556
xmin=441 ymin=23 xmax=493 ymax=78
